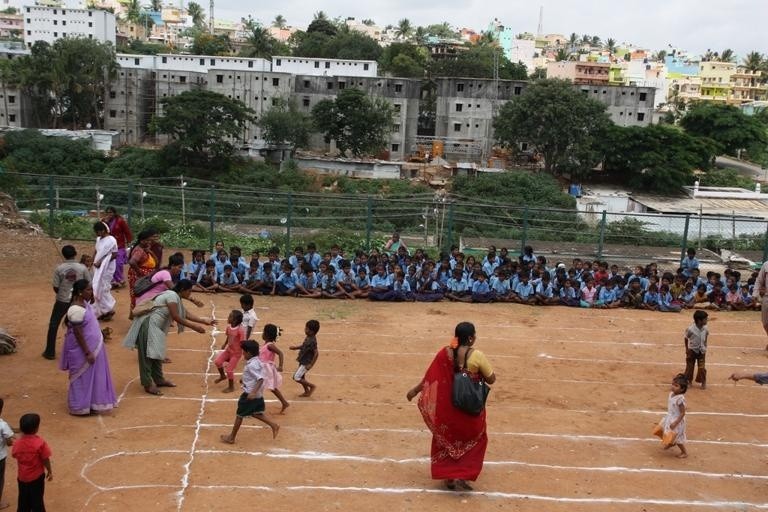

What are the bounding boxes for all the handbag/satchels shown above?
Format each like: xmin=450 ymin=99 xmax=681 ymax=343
xmin=131 ymin=293 xmax=168 ymax=315
xmin=453 ymin=348 xmax=491 ymax=414
xmin=133 ymin=268 xmax=163 ymax=297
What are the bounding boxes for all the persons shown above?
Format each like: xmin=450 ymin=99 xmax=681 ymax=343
xmin=653 ymin=373 xmax=689 ymax=457
xmin=752 ymin=261 xmax=768 ymax=349
xmin=43 ymin=208 xmax=219 ymax=413
xmin=174 ymin=248 xmax=768 ymax=312
xmin=0 ymin=398 xmax=18 ymax=509
xmin=11 ymin=413 xmax=53 ymax=512
xmin=214 ymin=296 xmax=320 ymax=444
xmin=729 ymin=372 xmax=768 ymax=384
xmin=407 ymin=322 xmax=496 ymax=489
xmin=685 ymin=311 xmax=709 ymax=389
